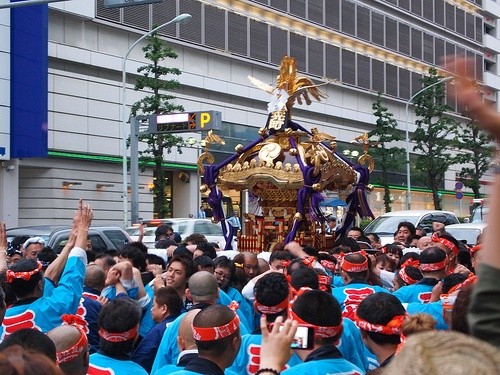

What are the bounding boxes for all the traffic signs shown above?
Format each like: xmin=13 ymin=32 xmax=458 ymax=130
xmin=155 ymin=111 xmax=225 ymax=134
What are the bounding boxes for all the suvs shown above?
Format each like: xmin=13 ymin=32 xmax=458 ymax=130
xmin=471 ymin=205 xmax=489 ymax=223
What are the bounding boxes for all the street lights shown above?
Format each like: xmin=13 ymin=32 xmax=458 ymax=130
xmin=405 ymin=75 xmax=458 ymax=211
xmin=122 ymin=12 xmax=191 ymax=229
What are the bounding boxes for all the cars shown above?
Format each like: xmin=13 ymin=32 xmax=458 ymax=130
xmin=440 ymin=224 xmax=487 ymax=246
xmin=5 ymin=218 xmax=235 ymax=274
xmin=363 ymin=212 xmax=460 ymax=251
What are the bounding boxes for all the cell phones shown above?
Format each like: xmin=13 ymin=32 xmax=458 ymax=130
xmin=271 ymin=325 xmax=314 ymax=350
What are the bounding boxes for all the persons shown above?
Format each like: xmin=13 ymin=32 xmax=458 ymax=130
xmin=0 ymin=86 xmax=500 ymax=375
